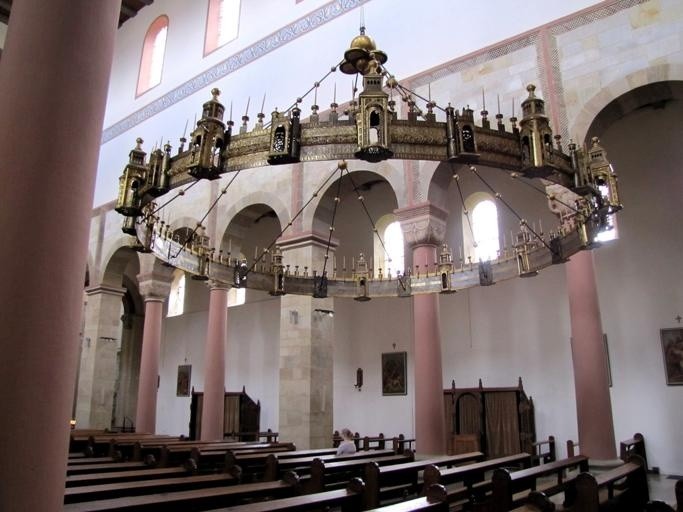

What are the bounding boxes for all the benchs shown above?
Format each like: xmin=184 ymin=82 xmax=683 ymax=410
xmin=63 ymin=422 xmax=649 ymax=512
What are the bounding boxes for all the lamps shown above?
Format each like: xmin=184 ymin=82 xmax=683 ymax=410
xmin=114 ymin=0 xmax=622 ymax=302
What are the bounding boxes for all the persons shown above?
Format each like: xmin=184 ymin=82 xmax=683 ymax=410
xmin=334 ymin=428 xmax=357 ymax=456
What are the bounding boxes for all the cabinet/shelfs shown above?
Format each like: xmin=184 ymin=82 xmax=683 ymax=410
xmin=442 ymin=377 xmax=537 ymax=458
xmin=189 ymin=385 xmax=259 ymax=441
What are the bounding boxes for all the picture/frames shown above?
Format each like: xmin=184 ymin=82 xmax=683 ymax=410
xmin=570 ymin=328 xmax=683 ymax=386
xmin=380 ymin=351 xmax=406 ymax=396
xmin=175 ymin=365 xmax=190 ymax=397
